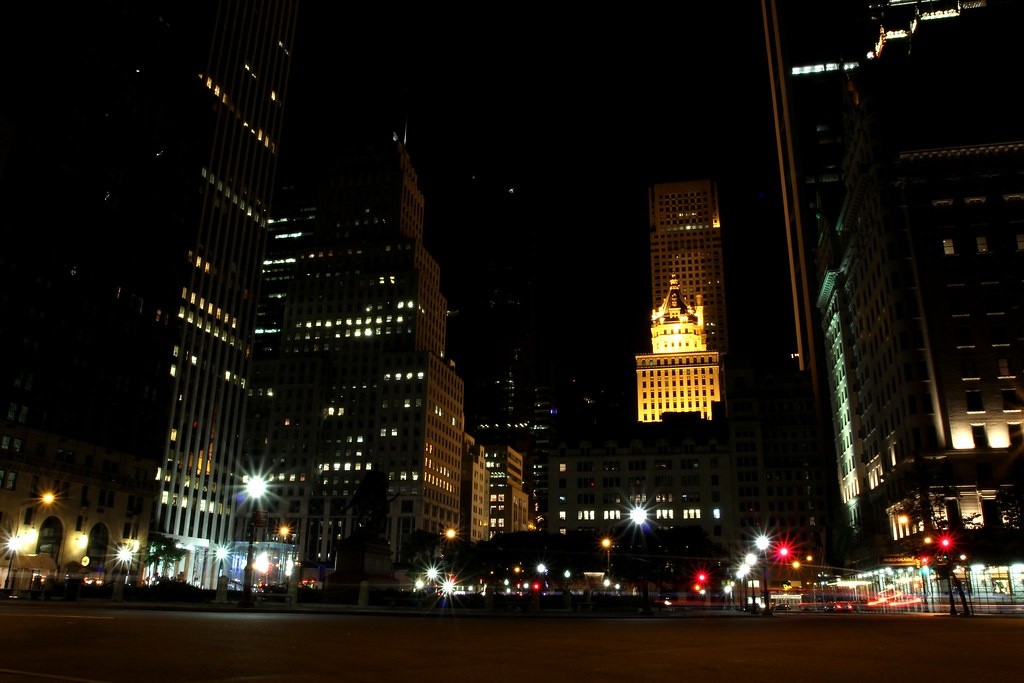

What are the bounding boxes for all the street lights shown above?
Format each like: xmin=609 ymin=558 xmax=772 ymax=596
xmin=922 ymin=528 xmax=968 ymax=618
xmin=0 ymin=490 xmax=132 ymax=601
xmin=721 ymin=530 xmax=773 ymax=618
xmin=239 ymin=474 xmax=267 ymax=608
